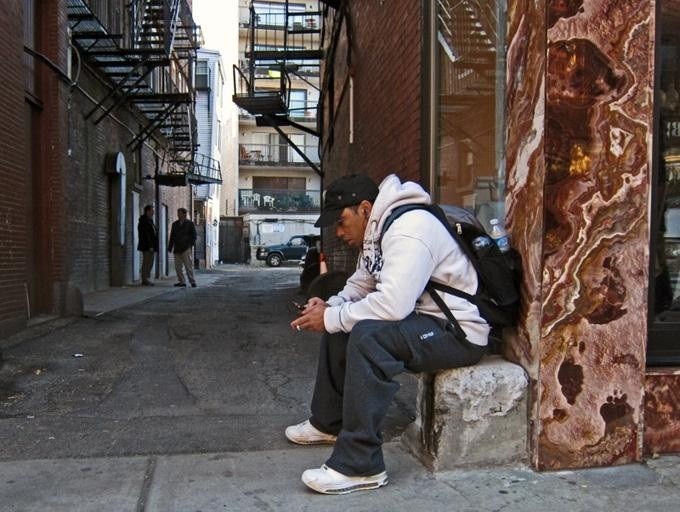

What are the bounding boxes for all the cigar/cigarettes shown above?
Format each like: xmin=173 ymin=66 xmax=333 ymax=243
xmin=295 ymin=324 xmax=302 ymax=332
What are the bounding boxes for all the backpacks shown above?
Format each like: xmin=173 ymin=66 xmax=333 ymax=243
xmin=378 ymin=201 xmax=523 ymax=339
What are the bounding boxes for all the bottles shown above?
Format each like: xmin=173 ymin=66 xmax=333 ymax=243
xmin=489 ymin=218 xmax=510 ymax=255
xmin=469 ymin=227 xmax=518 ymax=304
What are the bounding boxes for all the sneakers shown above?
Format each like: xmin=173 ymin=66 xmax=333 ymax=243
xmin=301 ymin=463 xmax=389 ymax=494
xmin=284 ymin=419 xmax=338 ymax=444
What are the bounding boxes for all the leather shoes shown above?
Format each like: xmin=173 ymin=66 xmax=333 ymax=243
xmin=174 ymin=283 xmax=195 ymax=287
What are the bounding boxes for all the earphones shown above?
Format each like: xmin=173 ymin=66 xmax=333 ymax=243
xmin=363 ymin=209 xmax=368 ymax=214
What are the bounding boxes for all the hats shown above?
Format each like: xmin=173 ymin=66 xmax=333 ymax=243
xmin=314 ymin=175 xmax=378 ymax=227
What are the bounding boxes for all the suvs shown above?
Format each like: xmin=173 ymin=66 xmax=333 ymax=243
xmin=256 ymin=235 xmax=321 ymax=267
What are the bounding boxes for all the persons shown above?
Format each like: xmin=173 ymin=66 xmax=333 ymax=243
xmin=286 ymin=173 xmax=492 ymax=494
xmin=168 ymin=208 xmax=197 ymax=288
xmin=137 ymin=205 xmax=159 ymax=286
